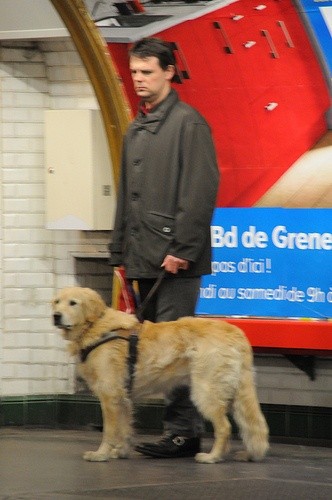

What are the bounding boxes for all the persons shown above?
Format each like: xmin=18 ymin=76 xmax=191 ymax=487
xmin=108 ymin=35 xmax=218 ymax=459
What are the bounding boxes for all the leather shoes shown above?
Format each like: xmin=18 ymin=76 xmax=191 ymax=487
xmin=134 ymin=433 xmax=201 ymax=459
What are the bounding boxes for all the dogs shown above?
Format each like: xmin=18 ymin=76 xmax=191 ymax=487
xmin=50 ymin=287 xmax=270 ymax=464
